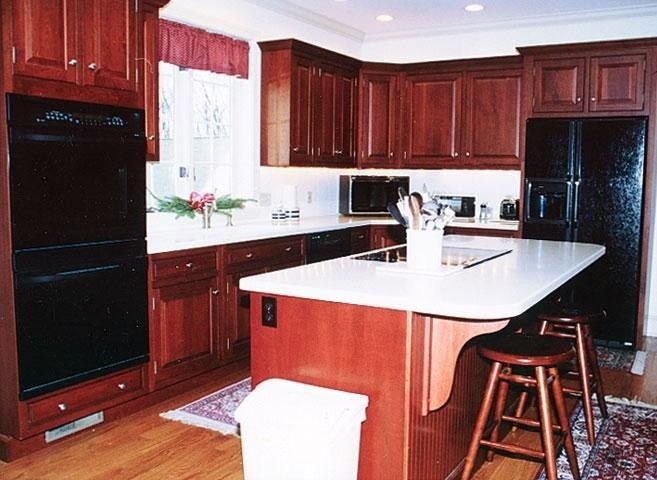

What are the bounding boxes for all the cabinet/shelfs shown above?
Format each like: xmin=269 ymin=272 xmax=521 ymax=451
xmin=517 ymin=40 xmax=656 ymax=120
xmin=0 ymin=1 xmax=171 ymax=162
xmin=402 ymin=55 xmax=523 ymax=168
xmin=219 ymin=234 xmax=303 ymax=367
xmin=256 ymin=37 xmax=360 ymax=168
xmin=0 ymin=366 xmax=148 ymax=463
xmin=148 ymin=249 xmax=220 ymax=393
xmin=359 ymin=62 xmax=402 ymax=170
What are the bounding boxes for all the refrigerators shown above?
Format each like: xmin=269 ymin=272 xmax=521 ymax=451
xmin=521 ymin=115 xmax=650 ymax=347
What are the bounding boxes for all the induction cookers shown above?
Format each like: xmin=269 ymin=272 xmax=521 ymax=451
xmin=351 ymin=245 xmax=512 ymax=270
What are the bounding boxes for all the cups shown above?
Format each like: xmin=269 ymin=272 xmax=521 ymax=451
xmin=406 ymin=228 xmax=444 ymax=269
xmin=285 ymin=209 xmax=300 ymax=224
xmin=271 ymin=210 xmax=286 ymax=223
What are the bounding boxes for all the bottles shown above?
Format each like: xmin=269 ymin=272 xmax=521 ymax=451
xmin=478 ymin=204 xmax=486 ymax=218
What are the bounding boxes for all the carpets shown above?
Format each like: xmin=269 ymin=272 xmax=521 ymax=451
xmin=533 ymin=392 xmax=657 ymax=480
xmin=159 ymin=376 xmax=252 ymax=439
xmin=565 ymin=344 xmax=636 ymax=373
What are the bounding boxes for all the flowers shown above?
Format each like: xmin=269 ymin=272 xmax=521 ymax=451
xmin=147 ymin=189 xmax=259 ymax=219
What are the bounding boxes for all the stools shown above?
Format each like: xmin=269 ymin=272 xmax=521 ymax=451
xmin=512 ymin=307 xmax=607 ymax=445
xmin=461 ymin=334 xmax=581 ymax=480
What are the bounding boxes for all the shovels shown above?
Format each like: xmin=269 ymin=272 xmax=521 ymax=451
xmin=387 ymin=201 xmax=408 ymax=229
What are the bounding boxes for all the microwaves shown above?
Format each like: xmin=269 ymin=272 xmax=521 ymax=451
xmin=339 ymin=175 xmax=409 ymax=216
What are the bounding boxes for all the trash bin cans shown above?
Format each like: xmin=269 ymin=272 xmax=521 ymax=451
xmin=233 ymin=378 xmax=369 ymax=480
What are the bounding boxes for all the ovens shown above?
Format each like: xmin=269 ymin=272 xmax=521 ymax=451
xmin=5 ymin=91 xmax=150 ymax=401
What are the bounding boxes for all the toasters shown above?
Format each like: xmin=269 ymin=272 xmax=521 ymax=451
xmin=500 ymin=199 xmax=519 ymax=220
